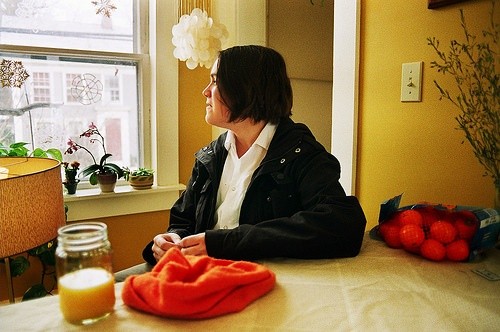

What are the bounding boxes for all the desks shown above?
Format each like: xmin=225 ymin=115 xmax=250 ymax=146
xmin=0 ymin=230 xmax=500 ymax=332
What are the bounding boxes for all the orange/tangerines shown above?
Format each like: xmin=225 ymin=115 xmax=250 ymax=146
xmin=379 ymin=209 xmax=477 ymax=259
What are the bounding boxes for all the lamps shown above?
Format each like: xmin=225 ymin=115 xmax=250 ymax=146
xmin=0 ymin=156 xmax=66 ymax=305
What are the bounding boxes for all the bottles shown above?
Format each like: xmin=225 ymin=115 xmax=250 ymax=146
xmin=55 ymin=221 xmax=116 ymax=324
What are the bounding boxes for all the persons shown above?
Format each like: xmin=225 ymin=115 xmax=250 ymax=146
xmin=113 ymin=44 xmax=367 ymax=282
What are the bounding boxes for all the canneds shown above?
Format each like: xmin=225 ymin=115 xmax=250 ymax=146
xmin=56 ymin=222 xmax=116 ymax=326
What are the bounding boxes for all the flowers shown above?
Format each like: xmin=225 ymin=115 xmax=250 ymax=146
xmin=61 ymin=161 xmax=80 ymax=186
xmin=64 ymin=122 xmax=125 ymax=185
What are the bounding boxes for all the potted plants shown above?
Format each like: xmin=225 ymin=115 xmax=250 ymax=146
xmin=123 ymin=166 xmax=155 ymax=190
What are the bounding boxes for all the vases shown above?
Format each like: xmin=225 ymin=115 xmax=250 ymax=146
xmin=95 ymin=172 xmax=117 ymax=192
xmin=64 ymin=181 xmax=78 ymax=195
